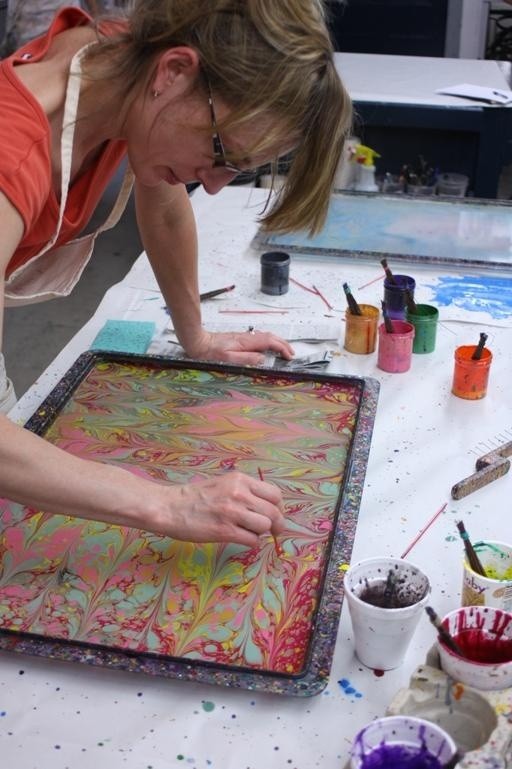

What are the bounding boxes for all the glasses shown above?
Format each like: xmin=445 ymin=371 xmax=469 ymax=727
xmin=200 ymin=66 xmax=259 ymax=178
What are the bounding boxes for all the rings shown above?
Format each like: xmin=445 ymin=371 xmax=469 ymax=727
xmin=248 ymin=325 xmax=255 ymax=335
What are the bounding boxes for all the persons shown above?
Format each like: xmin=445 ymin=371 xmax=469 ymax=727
xmin=0 ymin=0 xmax=98 ymax=59
xmin=0 ymin=0 xmax=356 ymax=550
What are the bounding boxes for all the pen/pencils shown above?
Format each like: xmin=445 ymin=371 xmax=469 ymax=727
xmin=493 ymin=91 xmax=508 ymax=99
xmin=384 ymin=162 xmax=436 ymax=187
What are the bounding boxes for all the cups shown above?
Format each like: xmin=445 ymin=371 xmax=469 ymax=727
xmin=258 ymin=173 xmax=287 ymax=189
xmin=374 ymin=170 xmax=469 ymax=198
xmin=403 ymin=304 xmax=439 ymax=356
xmin=436 ymin=604 xmax=512 ymax=690
xmin=377 ymin=320 xmax=418 ymax=373
xmin=343 ymin=304 xmax=379 ymax=356
xmin=459 ymin=541 xmax=512 ymax=612
xmin=346 ymin=715 xmax=461 ymax=769
xmin=343 ymin=555 xmax=433 ymax=673
xmin=384 ymin=274 xmax=416 ymax=319
xmin=452 ymin=344 xmax=493 ymax=402
xmin=260 ymin=250 xmax=291 ymax=297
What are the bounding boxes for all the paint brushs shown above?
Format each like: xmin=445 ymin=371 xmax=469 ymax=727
xmin=472 ymin=333 xmax=488 ymax=360
xmin=218 ymin=308 xmax=289 ymax=313
xmin=343 ymin=258 xmax=418 ymax=334
xmin=289 ymin=277 xmax=334 ymax=310
xmin=425 ymin=607 xmax=464 ymax=658
xmin=401 ymin=503 xmax=448 ymax=559
xmin=258 ymin=467 xmax=280 ymax=556
xmin=163 ymin=284 xmax=235 ymax=310
xmin=457 ymin=521 xmax=487 ymax=577
